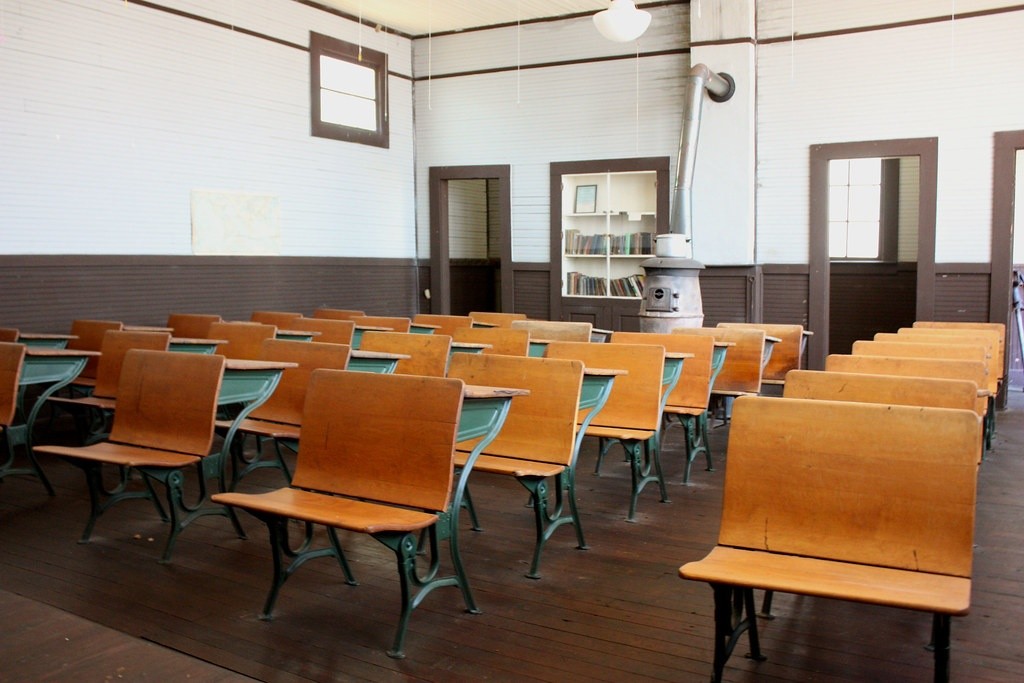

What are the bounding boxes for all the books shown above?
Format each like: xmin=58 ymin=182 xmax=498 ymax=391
xmin=564 ymin=227 xmax=657 ymax=256
xmin=566 ymin=270 xmax=646 ymax=297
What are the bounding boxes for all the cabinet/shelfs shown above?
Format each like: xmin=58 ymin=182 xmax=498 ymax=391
xmin=550 ymin=156 xmax=671 ymax=332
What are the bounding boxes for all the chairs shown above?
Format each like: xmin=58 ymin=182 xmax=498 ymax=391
xmin=0 ymin=309 xmax=805 ymax=557
xmin=677 ymin=320 xmax=1008 ymax=683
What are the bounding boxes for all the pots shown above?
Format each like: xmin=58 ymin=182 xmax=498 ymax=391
xmin=653 ymin=231 xmax=691 ymax=257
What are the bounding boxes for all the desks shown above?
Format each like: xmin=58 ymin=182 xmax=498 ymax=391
xmin=2 ymin=322 xmax=815 ymax=657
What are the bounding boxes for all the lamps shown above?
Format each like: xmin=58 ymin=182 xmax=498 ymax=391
xmin=593 ymin=0 xmax=651 ymax=42
